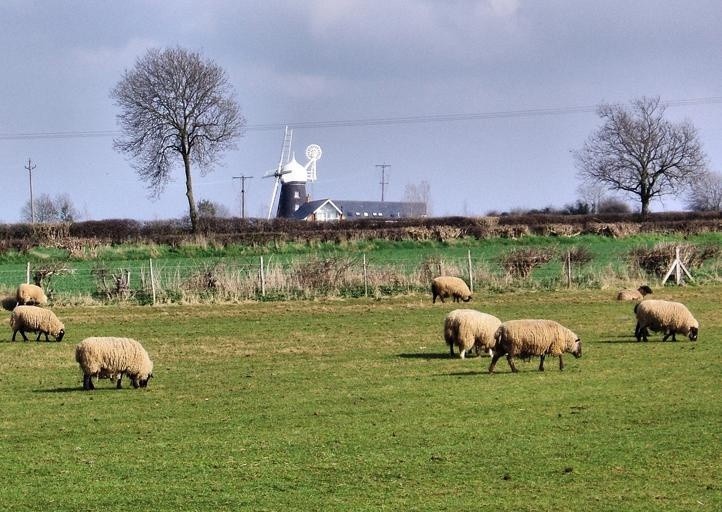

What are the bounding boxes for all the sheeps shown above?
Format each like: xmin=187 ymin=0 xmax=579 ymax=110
xmin=489 ymin=318 xmax=582 ymax=375
xmin=443 ymin=308 xmax=503 ymax=359
xmin=615 ymin=285 xmax=653 ymax=301
xmin=10 ymin=304 xmax=65 ymax=342
xmin=73 ymin=336 xmax=154 ymax=391
xmin=431 ymin=275 xmax=474 ymax=303
xmin=634 ymin=300 xmax=700 ymax=343
xmin=15 ymin=283 xmax=49 ymax=307
